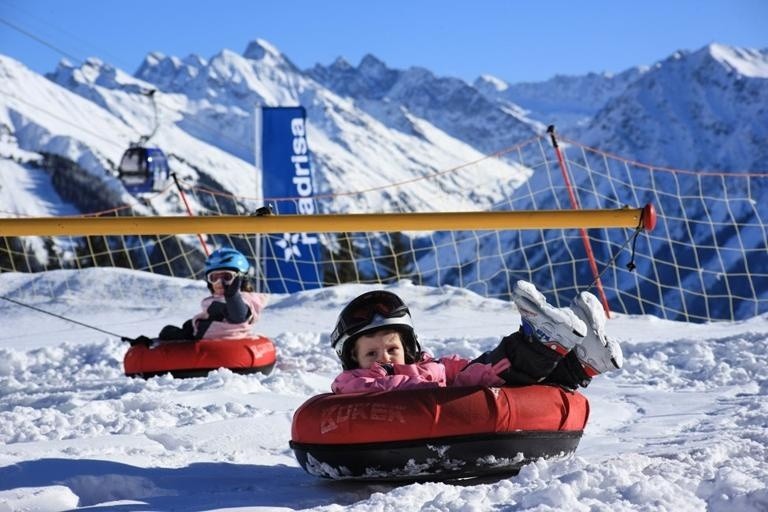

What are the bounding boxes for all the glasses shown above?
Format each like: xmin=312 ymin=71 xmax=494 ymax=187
xmin=207 ymin=270 xmax=238 ymax=284
xmin=330 ymin=291 xmax=411 ymax=349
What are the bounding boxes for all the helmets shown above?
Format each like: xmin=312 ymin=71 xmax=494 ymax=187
xmin=204 ymin=247 xmax=249 ymax=275
xmin=334 ymin=291 xmax=421 ymax=370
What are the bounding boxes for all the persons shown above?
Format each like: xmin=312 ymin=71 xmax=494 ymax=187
xmin=120 ymin=245 xmax=269 ymax=345
xmin=324 ymin=276 xmax=624 ymax=401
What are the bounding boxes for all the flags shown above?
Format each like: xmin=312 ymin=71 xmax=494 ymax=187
xmin=256 ymin=99 xmax=327 ymax=293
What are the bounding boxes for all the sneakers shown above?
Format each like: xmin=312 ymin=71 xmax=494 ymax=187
xmin=570 ymin=291 xmax=624 ymax=377
xmin=512 ymin=279 xmax=588 ymax=356
